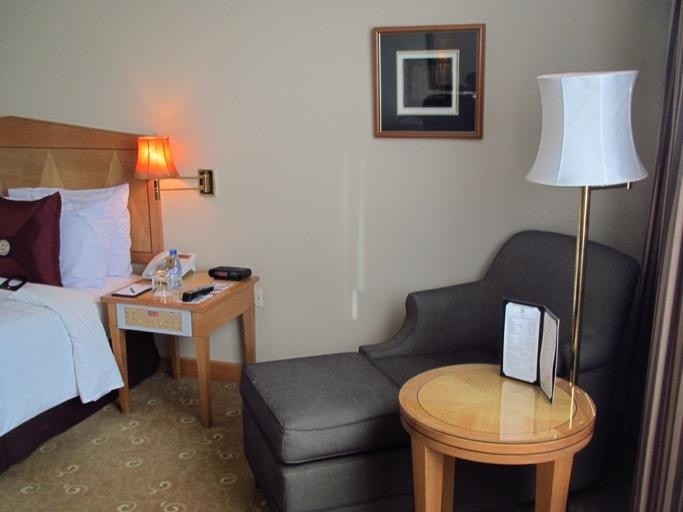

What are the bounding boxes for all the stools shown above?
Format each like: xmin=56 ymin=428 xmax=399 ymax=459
xmin=236 ymin=352 xmax=408 ymax=511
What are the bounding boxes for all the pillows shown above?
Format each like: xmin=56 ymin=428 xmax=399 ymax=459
xmin=0 ymin=170 xmax=136 ymax=291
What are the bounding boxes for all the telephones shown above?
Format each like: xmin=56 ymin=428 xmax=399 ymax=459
xmin=142 ymin=249 xmax=196 ymax=283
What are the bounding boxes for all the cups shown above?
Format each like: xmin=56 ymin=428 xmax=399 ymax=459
xmin=152 ymin=276 xmax=169 ymax=298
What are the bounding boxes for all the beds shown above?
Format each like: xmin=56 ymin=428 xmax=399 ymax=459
xmin=1 ymin=114 xmax=163 ymax=473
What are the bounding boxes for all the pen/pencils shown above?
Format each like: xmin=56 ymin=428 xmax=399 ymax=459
xmin=130 ymin=288 xmax=135 ymax=294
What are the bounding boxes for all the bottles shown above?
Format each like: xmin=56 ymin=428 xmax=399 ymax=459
xmin=166 ymin=249 xmax=183 ymax=297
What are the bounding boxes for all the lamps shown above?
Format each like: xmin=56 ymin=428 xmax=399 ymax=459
xmin=522 ymin=65 xmax=651 ymax=394
xmin=131 ymin=133 xmax=215 ymax=200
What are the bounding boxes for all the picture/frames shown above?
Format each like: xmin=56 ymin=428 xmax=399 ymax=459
xmin=373 ymin=21 xmax=486 ymax=140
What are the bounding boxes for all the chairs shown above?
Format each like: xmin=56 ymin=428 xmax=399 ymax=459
xmin=361 ymin=229 xmax=640 ymax=512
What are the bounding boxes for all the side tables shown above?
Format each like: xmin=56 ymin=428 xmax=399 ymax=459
xmin=394 ymin=364 xmax=596 ymax=512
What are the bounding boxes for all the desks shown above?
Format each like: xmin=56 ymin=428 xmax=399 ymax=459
xmin=97 ymin=272 xmax=261 ymax=430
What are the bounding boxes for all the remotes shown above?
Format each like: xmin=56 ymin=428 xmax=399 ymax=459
xmin=182 ymin=284 xmax=214 ymax=302
